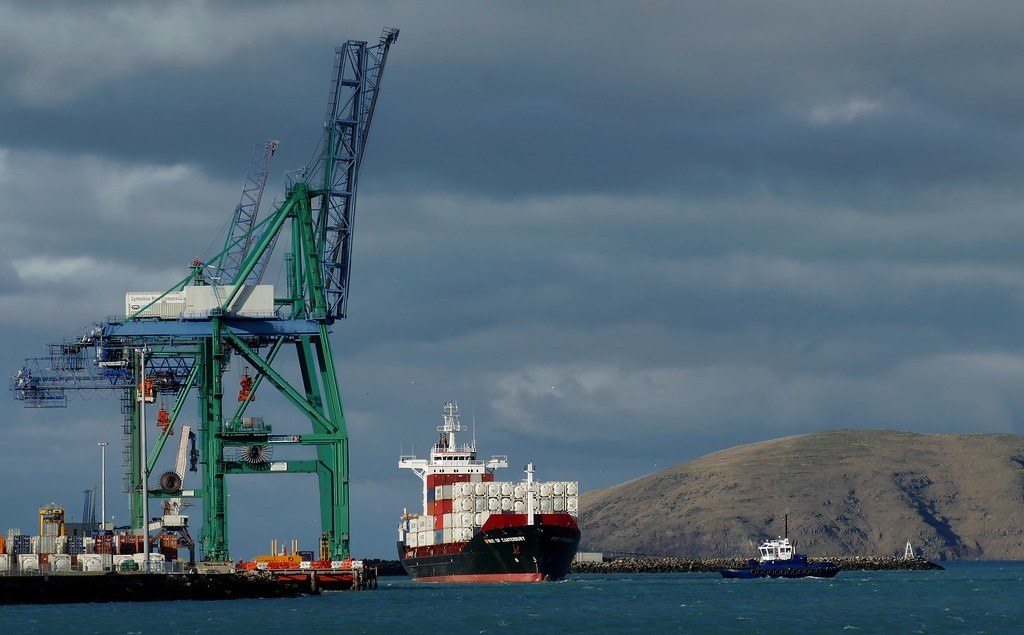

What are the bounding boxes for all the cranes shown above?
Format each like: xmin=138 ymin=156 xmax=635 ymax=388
xmin=10 ymin=140 xmax=277 ymax=564
xmin=148 ymin=425 xmax=198 ymax=566
xmin=89 ymin=27 xmax=398 ymax=562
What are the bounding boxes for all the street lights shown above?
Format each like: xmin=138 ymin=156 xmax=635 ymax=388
xmin=97 ymin=442 xmax=109 ymax=529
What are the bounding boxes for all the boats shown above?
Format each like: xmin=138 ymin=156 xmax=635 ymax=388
xmin=719 ymin=513 xmax=843 ymax=579
xmin=397 ymin=402 xmax=581 ymax=582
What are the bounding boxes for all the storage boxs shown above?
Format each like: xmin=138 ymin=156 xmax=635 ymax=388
xmin=125 ymin=291 xmax=186 ymax=321
xmin=0 ymin=534 xmax=178 ymax=567
xmin=236 ymin=560 xmax=362 ymax=570
xmin=406 ymin=473 xmax=494 ymax=547
xmin=577 ymin=552 xmax=604 ymax=563
